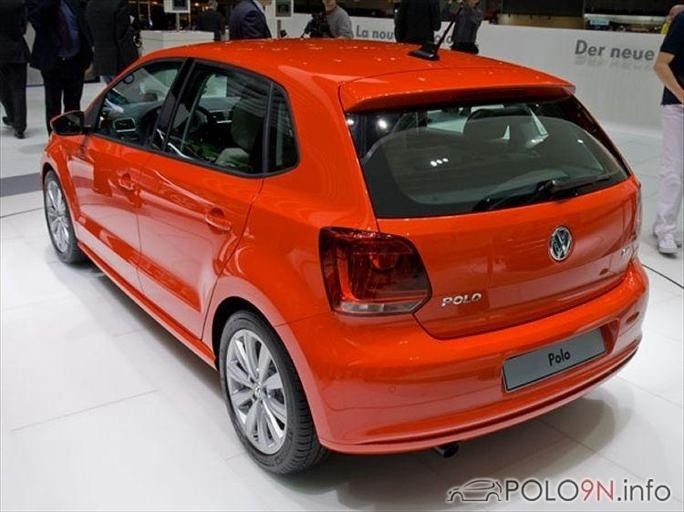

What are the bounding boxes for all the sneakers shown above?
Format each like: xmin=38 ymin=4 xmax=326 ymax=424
xmin=652 ymin=224 xmax=683 ymax=255
xmin=1 ymin=116 xmax=25 ymax=139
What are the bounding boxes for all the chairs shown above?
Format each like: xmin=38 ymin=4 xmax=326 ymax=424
xmin=215 ymin=95 xmax=295 ymax=172
xmin=458 ymin=105 xmax=542 ymax=165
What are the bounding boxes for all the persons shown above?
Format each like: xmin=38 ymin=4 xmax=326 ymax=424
xmin=660 ymin=4 xmax=683 ymax=36
xmin=0 ymin=1 xmax=499 ymax=139
xmin=650 ymin=11 xmax=683 ymax=256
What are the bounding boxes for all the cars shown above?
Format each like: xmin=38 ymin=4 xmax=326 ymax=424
xmin=38 ymin=32 xmax=653 ymax=476
